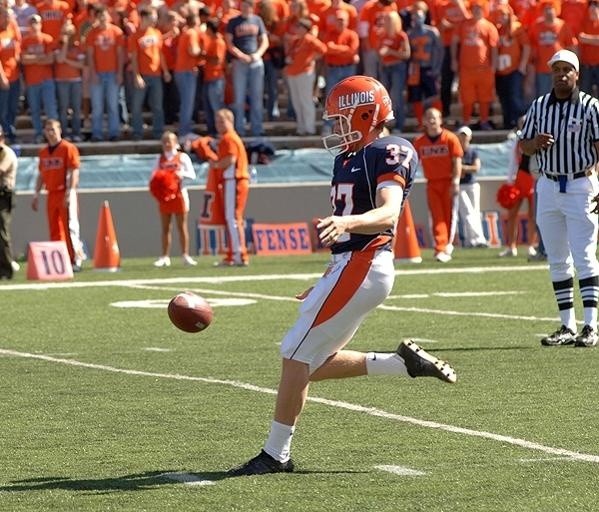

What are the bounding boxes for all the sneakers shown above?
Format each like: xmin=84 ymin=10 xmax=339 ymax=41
xmin=154 ymin=257 xmax=170 ymax=266
xmin=434 ymin=243 xmax=486 ymax=262
xmin=496 ymin=243 xmax=547 ymax=262
xmin=396 ymin=338 xmax=457 ymax=384
xmin=227 ymin=449 xmax=294 ymax=479
xmin=184 ymin=256 xmax=197 ymax=266
xmin=575 ymin=324 xmax=599 ymax=348
xmin=541 ymin=325 xmax=580 ymax=346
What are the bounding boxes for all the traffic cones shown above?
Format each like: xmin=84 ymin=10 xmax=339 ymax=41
xmin=91 ymin=199 xmax=122 ymax=272
xmin=393 ymin=201 xmax=422 ymax=265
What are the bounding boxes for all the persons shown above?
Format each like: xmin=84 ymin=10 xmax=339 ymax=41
xmin=1 ymin=1 xmax=598 ymax=278
xmin=224 ymin=75 xmax=459 ymax=478
xmin=512 ymin=48 xmax=599 ymax=347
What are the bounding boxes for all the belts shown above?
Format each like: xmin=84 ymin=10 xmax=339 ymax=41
xmin=545 ymin=171 xmax=586 ymax=181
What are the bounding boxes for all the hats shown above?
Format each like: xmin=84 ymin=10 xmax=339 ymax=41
xmin=547 ymin=49 xmax=580 ymax=72
xmin=457 ymin=126 xmax=472 ymax=136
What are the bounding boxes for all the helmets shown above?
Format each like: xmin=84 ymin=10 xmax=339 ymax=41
xmin=320 ymin=74 xmax=396 ymax=157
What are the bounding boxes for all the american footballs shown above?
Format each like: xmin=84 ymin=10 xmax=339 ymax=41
xmin=169 ymin=292 xmax=214 ymax=332
